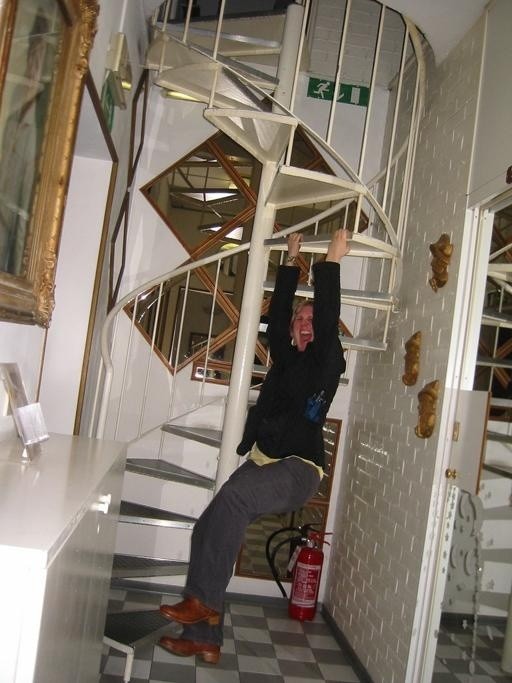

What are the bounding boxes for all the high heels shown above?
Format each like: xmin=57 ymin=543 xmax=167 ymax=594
xmin=160 ymin=594 xmax=220 ymax=626
xmin=159 ymin=635 xmax=221 ymax=663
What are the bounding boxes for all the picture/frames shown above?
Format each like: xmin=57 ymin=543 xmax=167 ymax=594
xmin=0 ymin=0 xmax=101 ymax=328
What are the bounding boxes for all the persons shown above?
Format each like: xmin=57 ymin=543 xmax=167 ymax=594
xmin=0 ymin=9 xmax=52 ymax=270
xmin=159 ymin=227 xmax=350 ymax=665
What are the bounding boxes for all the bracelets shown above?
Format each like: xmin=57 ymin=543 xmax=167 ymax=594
xmin=284 ymin=254 xmax=298 ymax=264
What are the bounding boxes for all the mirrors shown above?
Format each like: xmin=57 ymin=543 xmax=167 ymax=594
xmin=235 ymin=418 xmax=342 ymax=505
xmin=121 ymin=93 xmax=354 ymax=388
xmin=235 ymin=504 xmax=329 ymax=582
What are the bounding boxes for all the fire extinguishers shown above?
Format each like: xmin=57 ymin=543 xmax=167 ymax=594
xmin=271 ymin=532 xmax=333 ymax=622
xmin=265 ymin=522 xmax=321 ymax=578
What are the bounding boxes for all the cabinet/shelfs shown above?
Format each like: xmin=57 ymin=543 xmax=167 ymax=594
xmin=0 ymin=433 xmax=127 ymax=683
